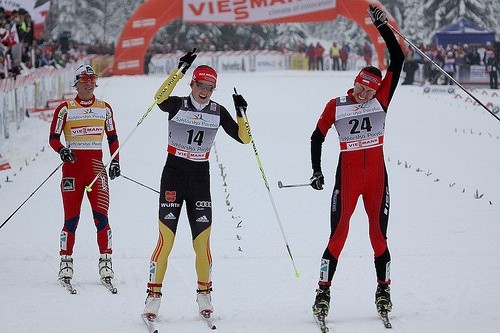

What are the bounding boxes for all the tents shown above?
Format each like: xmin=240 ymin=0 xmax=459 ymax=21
xmin=431 ymin=17 xmax=497 ymax=46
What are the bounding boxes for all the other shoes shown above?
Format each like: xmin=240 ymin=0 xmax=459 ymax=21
xmin=401 ymin=79 xmax=413 ymax=86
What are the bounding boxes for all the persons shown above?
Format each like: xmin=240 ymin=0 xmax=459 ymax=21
xmin=140 ymin=51 xmax=250 ymax=333
xmin=311 ymin=4 xmax=405 ymax=333
xmin=49 ymin=65 xmax=121 ymax=295
xmin=0 ymin=6 xmax=497 ymax=89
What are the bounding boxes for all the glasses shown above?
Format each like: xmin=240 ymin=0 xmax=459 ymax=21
xmin=195 ymin=80 xmax=215 ymax=93
xmin=76 ymin=74 xmax=99 ymax=83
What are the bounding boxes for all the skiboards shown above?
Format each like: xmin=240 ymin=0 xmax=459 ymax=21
xmin=140 ymin=312 xmax=217 ymax=333
xmin=57 ymin=277 xmax=118 ymax=295
xmin=314 ymin=313 xmax=392 ymax=333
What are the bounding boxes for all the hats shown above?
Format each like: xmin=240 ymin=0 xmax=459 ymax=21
xmin=18 ymin=9 xmax=28 ymax=15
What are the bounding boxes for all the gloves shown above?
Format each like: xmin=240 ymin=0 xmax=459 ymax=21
xmin=367 ymin=6 xmax=388 ymax=27
xmin=109 ymin=160 xmax=121 ymax=180
xmin=177 ymin=47 xmax=198 ymax=74
xmin=59 ymin=147 xmax=76 ymax=163
xmin=232 ymin=94 xmax=248 ymax=117
xmin=310 ymin=173 xmax=325 ymax=191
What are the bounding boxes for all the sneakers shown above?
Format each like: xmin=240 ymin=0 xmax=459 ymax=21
xmin=195 ymin=293 xmax=215 ymax=313
xmin=58 ymin=255 xmax=73 ymax=278
xmin=142 ymin=297 xmax=161 ymax=318
xmin=374 ymin=287 xmax=393 ymax=312
xmin=312 ymin=288 xmax=330 ymax=316
xmin=98 ymin=254 xmax=114 ymax=278
